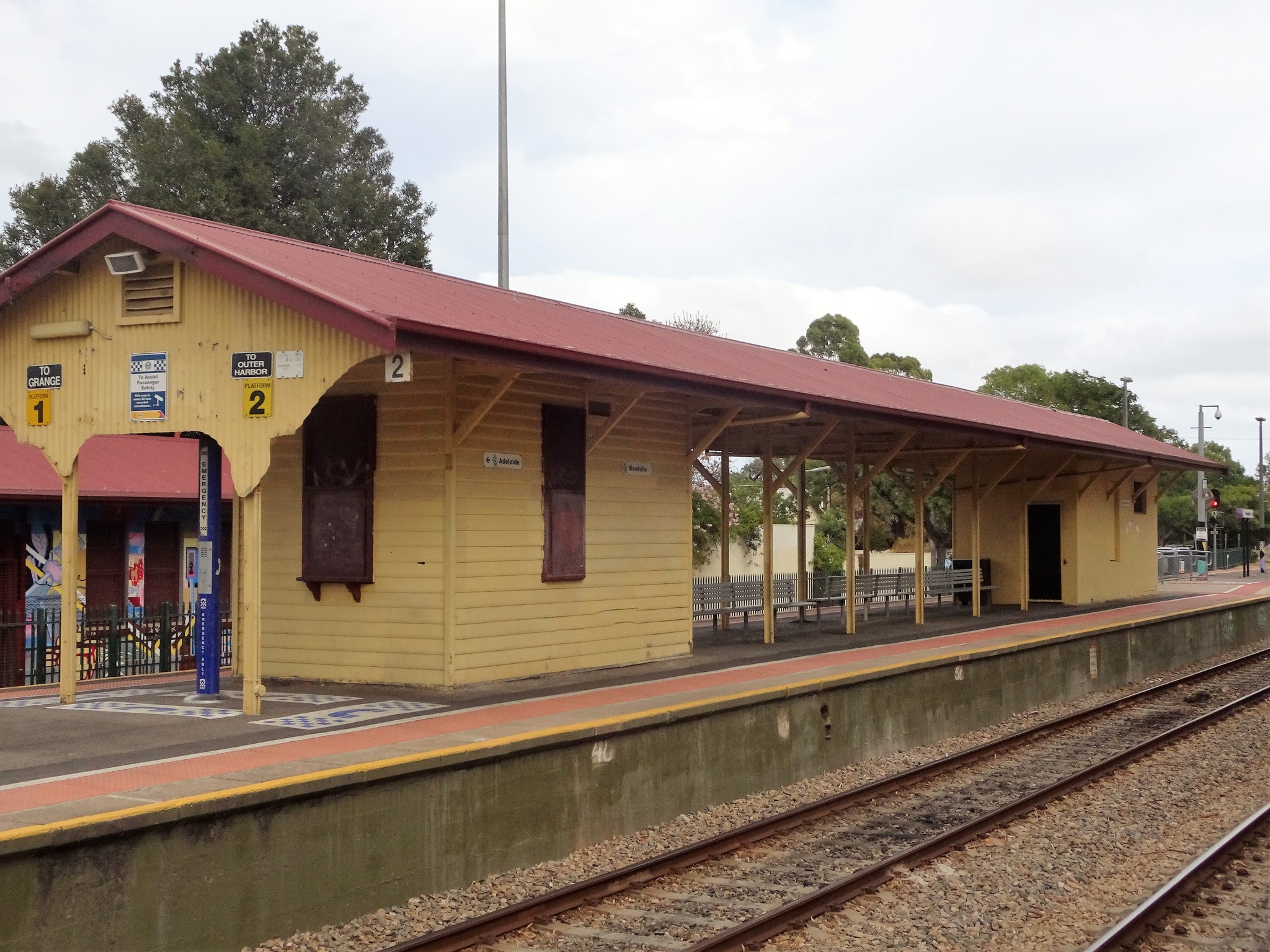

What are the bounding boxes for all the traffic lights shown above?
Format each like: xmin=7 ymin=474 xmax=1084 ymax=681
xmin=1211 ymin=488 xmax=1220 ymax=508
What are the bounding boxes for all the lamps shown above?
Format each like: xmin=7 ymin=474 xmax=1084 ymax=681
xmin=104 ymin=245 xmax=149 ymax=275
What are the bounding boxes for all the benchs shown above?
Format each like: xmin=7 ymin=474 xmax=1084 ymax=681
xmin=919 ymin=568 xmax=998 ymax=613
xmin=695 ymin=579 xmax=820 ymax=633
xmin=808 ymin=568 xmax=915 ymax=624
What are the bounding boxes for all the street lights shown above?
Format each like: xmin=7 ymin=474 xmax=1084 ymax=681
xmin=1119 ymin=377 xmax=1133 ymax=429
xmin=1197 ymin=404 xmax=1222 ymax=555
xmin=1254 ymin=416 xmax=1267 ymax=529
